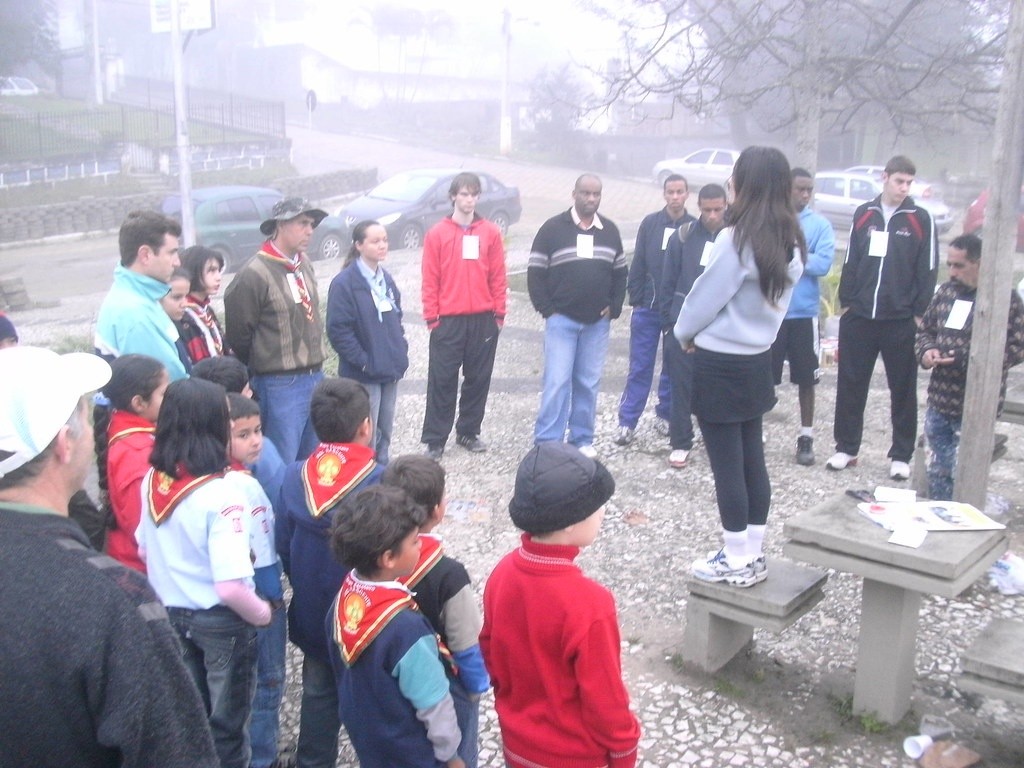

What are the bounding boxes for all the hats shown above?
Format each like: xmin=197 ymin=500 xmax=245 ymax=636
xmin=259 ymin=196 xmax=328 ymax=235
xmin=509 ymin=442 xmax=615 ymax=534
xmin=0 ymin=317 xmax=18 ymax=341
xmin=0 ymin=346 xmax=112 ymax=477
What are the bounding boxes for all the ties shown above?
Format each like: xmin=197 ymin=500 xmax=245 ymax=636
xmin=189 ymin=296 xmax=223 ymax=357
xmin=260 ymin=239 xmax=315 ymax=323
xmin=104 ymin=410 xmax=154 ymax=449
xmin=301 ymin=441 xmax=377 ymax=519
xmin=224 ymin=459 xmax=253 ymax=473
xmin=145 ymin=457 xmax=224 ymax=527
xmin=332 ymin=570 xmax=459 ymax=678
xmin=393 ymin=533 xmax=449 ymax=590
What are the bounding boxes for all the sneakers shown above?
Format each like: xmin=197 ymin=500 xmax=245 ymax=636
xmin=613 ymin=424 xmax=632 ymax=445
xmin=655 ymin=417 xmax=671 ymax=435
xmin=797 ymin=435 xmax=816 ymax=465
xmin=826 ymin=453 xmax=858 ymax=469
xmin=456 ymin=434 xmax=487 ymax=452
xmin=423 ymin=443 xmax=443 ymax=462
xmin=669 ymin=448 xmax=692 ymax=467
xmin=690 ymin=550 xmax=768 ymax=586
xmin=890 ymin=460 xmax=911 ymax=479
xmin=578 ymin=445 xmax=597 ymax=459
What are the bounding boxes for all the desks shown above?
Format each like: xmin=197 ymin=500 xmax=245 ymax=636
xmin=781 ymin=486 xmax=1009 ymax=726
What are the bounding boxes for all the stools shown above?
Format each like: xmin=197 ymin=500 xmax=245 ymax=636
xmin=684 ymin=551 xmax=829 ymax=672
xmin=957 ymin=620 xmax=1024 ymax=707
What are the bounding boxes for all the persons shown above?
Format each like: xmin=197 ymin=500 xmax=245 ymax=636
xmin=770 ymin=166 xmax=835 ymax=466
xmin=224 ymin=196 xmax=329 ymax=466
xmin=381 ymin=453 xmax=492 ymax=768
xmin=826 ymin=154 xmax=938 ymax=479
xmin=613 ymin=174 xmax=698 ymax=445
xmin=326 ymin=220 xmax=408 ymax=465
xmin=421 ymin=172 xmax=506 ymax=462
xmin=658 ymin=184 xmax=727 ymax=468
xmin=225 ymin=391 xmax=299 ymax=768
xmin=273 ymin=375 xmax=385 ymax=768
xmin=0 ymin=207 xmax=234 ymax=384
xmin=672 ymin=143 xmax=807 ymax=588
xmin=527 ymin=173 xmax=628 ymax=458
xmin=477 ymin=439 xmax=641 ymax=768
xmin=190 ymin=356 xmax=287 ymax=509
xmin=0 ymin=346 xmax=216 ymax=768
xmin=134 ymin=378 xmax=273 ymax=768
xmin=324 ymin=484 xmax=466 ymax=768
xmin=914 ymin=232 xmax=1024 ymax=502
xmin=93 ymin=354 xmax=171 ymax=575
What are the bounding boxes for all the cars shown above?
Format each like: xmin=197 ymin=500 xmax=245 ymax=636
xmin=813 ymin=172 xmax=953 ymax=236
xmin=0 ymin=76 xmax=38 ymax=97
xmin=652 ymin=147 xmax=741 ymax=195
xmin=962 ymin=186 xmax=1024 ymax=255
xmin=844 ymin=165 xmax=935 ymax=201
xmin=154 ymin=186 xmax=351 ymax=277
xmin=340 ymin=167 xmax=521 ymax=252
